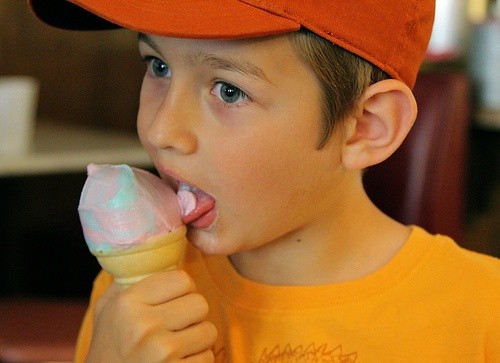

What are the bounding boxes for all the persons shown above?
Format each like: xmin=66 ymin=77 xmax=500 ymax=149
xmin=30 ymin=0 xmax=500 ymax=363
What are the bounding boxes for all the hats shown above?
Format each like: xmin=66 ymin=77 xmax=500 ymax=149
xmin=27 ymin=0 xmax=436 ymax=92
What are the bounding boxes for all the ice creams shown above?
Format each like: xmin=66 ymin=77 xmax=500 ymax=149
xmin=77 ymin=163 xmax=187 ymax=290
